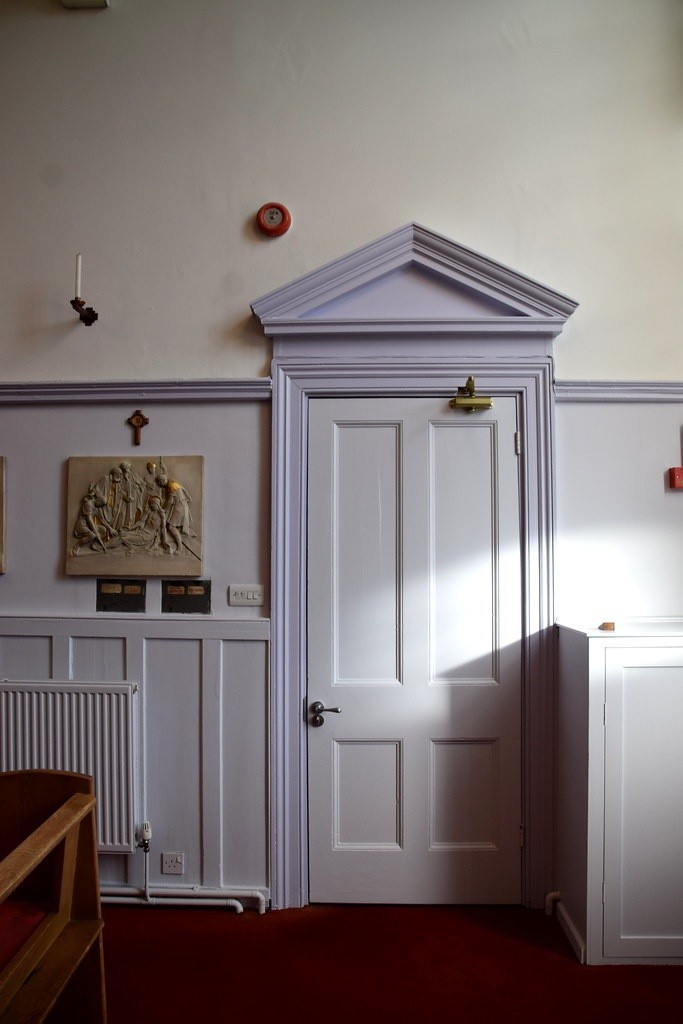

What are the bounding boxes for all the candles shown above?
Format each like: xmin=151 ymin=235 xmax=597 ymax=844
xmin=75 ymin=253 xmax=81 ymax=298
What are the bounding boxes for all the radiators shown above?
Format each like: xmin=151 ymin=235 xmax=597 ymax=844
xmin=0 ymin=679 xmax=139 ymax=854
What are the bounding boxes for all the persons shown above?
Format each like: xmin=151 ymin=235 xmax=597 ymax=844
xmin=72 ymin=462 xmax=194 ymax=557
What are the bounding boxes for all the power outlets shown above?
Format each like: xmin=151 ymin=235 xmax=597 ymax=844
xmin=163 ymin=850 xmax=186 ymax=875
